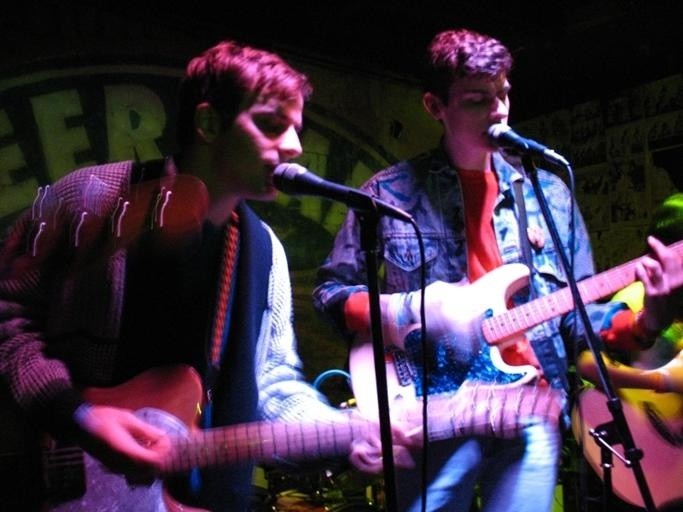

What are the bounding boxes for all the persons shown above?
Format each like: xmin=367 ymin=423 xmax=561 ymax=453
xmin=0 ymin=40 xmax=415 ymax=512
xmin=313 ymin=28 xmax=682 ymax=511
xmin=576 ymin=192 xmax=683 ymax=395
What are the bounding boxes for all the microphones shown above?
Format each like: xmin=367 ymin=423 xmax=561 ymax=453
xmin=273 ymin=162 xmax=414 ymax=224
xmin=488 ymin=122 xmax=568 ymax=167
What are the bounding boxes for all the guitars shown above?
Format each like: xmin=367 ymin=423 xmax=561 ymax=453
xmin=571 ymin=347 xmax=683 ymax=510
xmin=352 ymin=242 xmax=683 ymax=414
xmin=1 ymin=361 xmax=567 ymax=512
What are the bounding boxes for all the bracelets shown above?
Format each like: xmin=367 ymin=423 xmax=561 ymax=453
xmin=387 ymin=288 xmax=413 ymax=329
xmin=655 ymin=367 xmax=673 ymax=396
